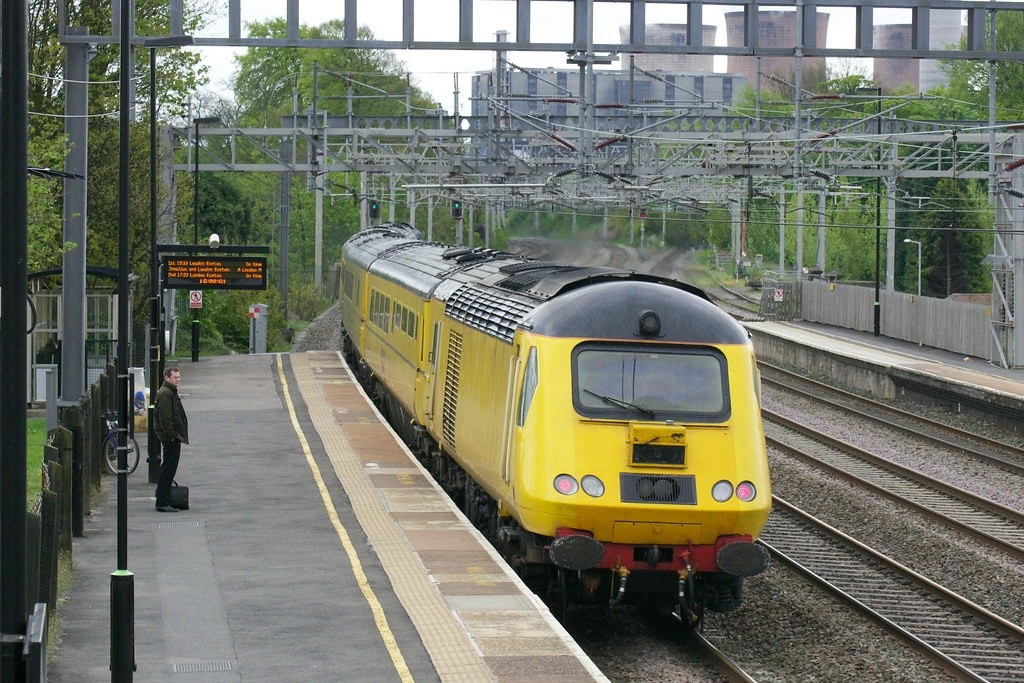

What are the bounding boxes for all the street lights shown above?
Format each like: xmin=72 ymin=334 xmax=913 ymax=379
xmin=142 ymin=35 xmax=195 ymax=481
xmin=904 ymin=239 xmax=921 ymax=296
xmin=857 ymin=87 xmax=882 ymax=336
xmin=192 ymin=115 xmax=222 ymax=361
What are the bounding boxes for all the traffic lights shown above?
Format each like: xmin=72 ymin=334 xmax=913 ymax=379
xmin=369 ymin=197 xmax=380 ymax=219
xmin=640 ymin=206 xmax=648 ymax=218
xmin=451 ymin=195 xmax=463 ymax=219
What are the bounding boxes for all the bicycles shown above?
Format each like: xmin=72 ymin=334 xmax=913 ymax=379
xmin=101 ymin=408 xmax=140 ymax=477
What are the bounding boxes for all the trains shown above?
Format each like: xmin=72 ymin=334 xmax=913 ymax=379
xmin=340 ymin=220 xmax=774 ymax=633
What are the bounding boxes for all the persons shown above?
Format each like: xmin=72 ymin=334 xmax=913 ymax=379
xmin=154 ymin=367 xmax=189 ymax=512
xmin=38 ymin=335 xmax=62 ymax=400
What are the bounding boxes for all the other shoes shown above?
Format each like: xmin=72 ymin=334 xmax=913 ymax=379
xmin=156 ymin=503 xmax=179 ymax=512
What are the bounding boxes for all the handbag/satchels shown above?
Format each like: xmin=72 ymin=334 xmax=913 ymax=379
xmin=168 ymin=479 xmax=189 ymax=510
